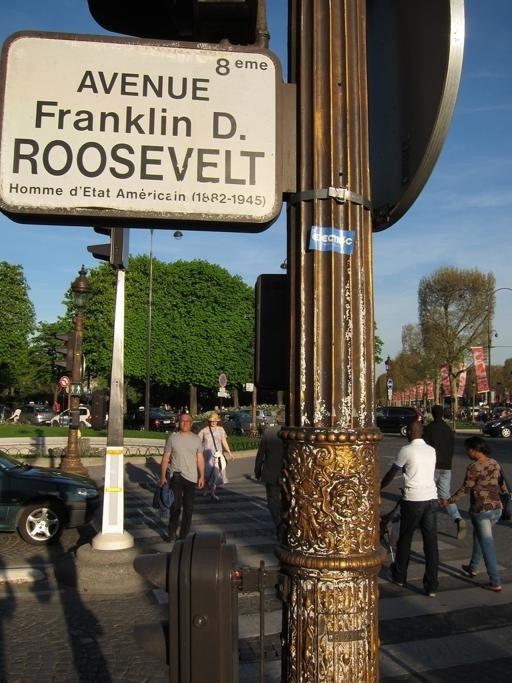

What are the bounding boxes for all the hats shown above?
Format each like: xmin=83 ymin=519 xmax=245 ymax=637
xmin=207 ymin=412 xmax=221 ymax=422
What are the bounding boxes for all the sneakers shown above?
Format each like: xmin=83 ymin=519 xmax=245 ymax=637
xmin=461 ymin=564 xmax=476 ymax=576
xmin=202 ymin=488 xmax=220 ymax=501
xmin=168 ymin=532 xmax=176 ymax=543
xmin=423 ymin=589 xmax=436 ymax=597
xmin=456 ymin=518 xmax=466 ymax=540
xmin=482 ymin=583 xmax=502 ymax=592
xmin=386 ymin=570 xmax=404 ymax=586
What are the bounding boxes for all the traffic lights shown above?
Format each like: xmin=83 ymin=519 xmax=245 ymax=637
xmin=64 ymin=383 xmax=82 ymax=396
xmin=132 ymin=529 xmax=288 ymax=683
xmin=51 ymin=331 xmax=75 ymax=374
xmin=89 ymin=227 xmax=130 ymax=268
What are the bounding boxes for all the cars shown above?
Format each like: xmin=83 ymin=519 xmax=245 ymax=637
xmin=482 ymin=414 xmax=512 ymax=438
xmin=19 ymin=403 xmax=92 ymax=429
xmin=192 ymin=409 xmax=273 ymax=436
xmin=377 ymin=406 xmax=425 ymax=437
xmin=0 ymin=450 xmax=100 ymax=546
xmin=124 ymin=408 xmax=176 ymax=432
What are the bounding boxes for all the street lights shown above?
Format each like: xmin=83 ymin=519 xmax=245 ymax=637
xmin=385 ymin=355 xmax=392 ymax=406
xmin=58 ymin=261 xmax=92 ymax=481
xmin=144 ymin=230 xmax=184 ymax=429
xmin=488 ymin=288 xmax=512 ymax=413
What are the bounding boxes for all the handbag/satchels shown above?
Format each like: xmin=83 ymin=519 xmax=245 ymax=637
xmin=218 ymin=454 xmax=228 ymax=472
xmin=498 ymin=492 xmax=512 ymax=521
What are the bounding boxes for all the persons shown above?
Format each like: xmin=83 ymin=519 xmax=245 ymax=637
xmin=380 ymin=421 xmax=439 ymax=597
xmin=162 ymin=412 xmax=205 ymax=543
xmin=198 ymin=412 xmax=234 ymax=501
xmin=439 ymin=437 xmax=509 ymax=591
xmin=422 ymin=404 xmax=467 ymax=540
